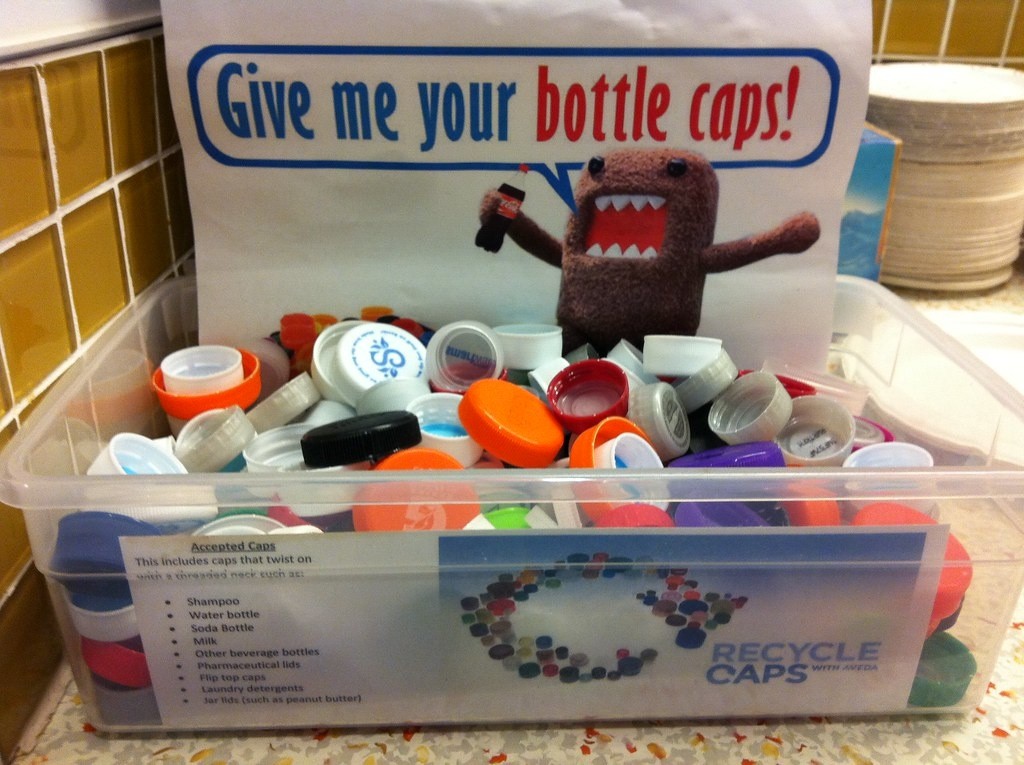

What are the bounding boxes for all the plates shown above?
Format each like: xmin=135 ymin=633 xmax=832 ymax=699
xmin=866 ymin=61 xmax=1024 ymax=291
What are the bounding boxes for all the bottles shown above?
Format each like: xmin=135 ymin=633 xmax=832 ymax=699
xmin=475 ymin=163 xmax=528 ymax=253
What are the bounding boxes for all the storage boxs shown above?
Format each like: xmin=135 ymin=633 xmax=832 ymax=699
xmin=0 ymin=275 xmax=1024 ymax=731
